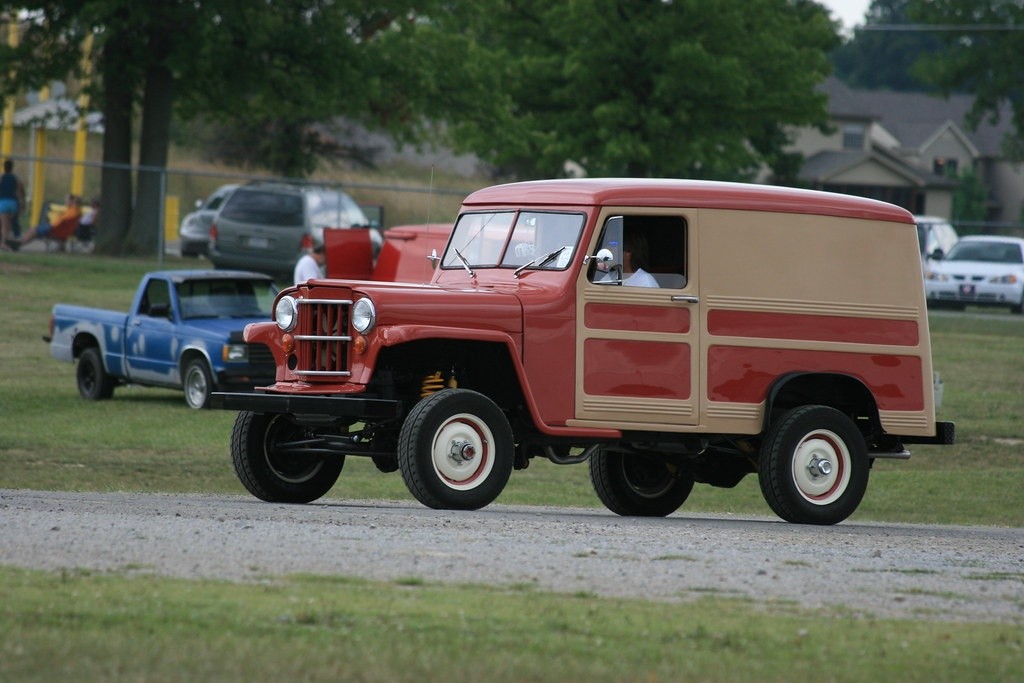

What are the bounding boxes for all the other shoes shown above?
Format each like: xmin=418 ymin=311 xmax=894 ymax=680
xmin=5 ymin=238 xmax=21 ymax=251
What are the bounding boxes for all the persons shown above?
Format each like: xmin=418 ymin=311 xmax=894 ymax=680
xmin=597 ymin=218 xmax=660 ymax=287
xmin=0 ymin=159 xmax=100 ymax=253
xmin=294 ymin=245 xmax=327 ymax=286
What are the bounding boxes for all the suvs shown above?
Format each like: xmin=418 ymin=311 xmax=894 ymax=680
xmin=206 ymin=180 xmax=386 ymax=286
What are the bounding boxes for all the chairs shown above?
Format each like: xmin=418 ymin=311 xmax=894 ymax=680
xmin=41 ymin=214 xmax=81 ymax=254
xmin=206 ymin=287 xmax=234 ymax=311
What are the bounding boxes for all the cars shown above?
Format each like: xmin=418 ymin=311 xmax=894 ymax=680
xmin=907 ymin=209 xmax=963 ymax=263
xmin=178 ymin=183 xmax=238 ymax=261
xmin=206 ymin=180 xmax=957 ymax=526
xmin=922 ymin=234 xmax=1024 ymax=312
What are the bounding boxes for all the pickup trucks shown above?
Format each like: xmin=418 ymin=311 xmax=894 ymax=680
xmin=46 ymin=270 xmax=282 ymax=413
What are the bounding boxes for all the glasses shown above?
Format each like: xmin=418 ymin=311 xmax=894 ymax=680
xmin=609 ymin=241 xmax=619 ymax=246
